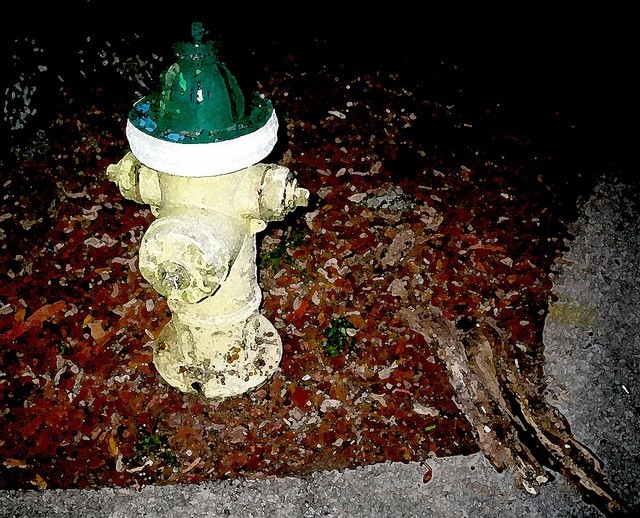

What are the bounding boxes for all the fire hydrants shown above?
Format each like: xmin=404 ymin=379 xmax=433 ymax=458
xmin=106 ymin=21 xmax=310 ymax=405
